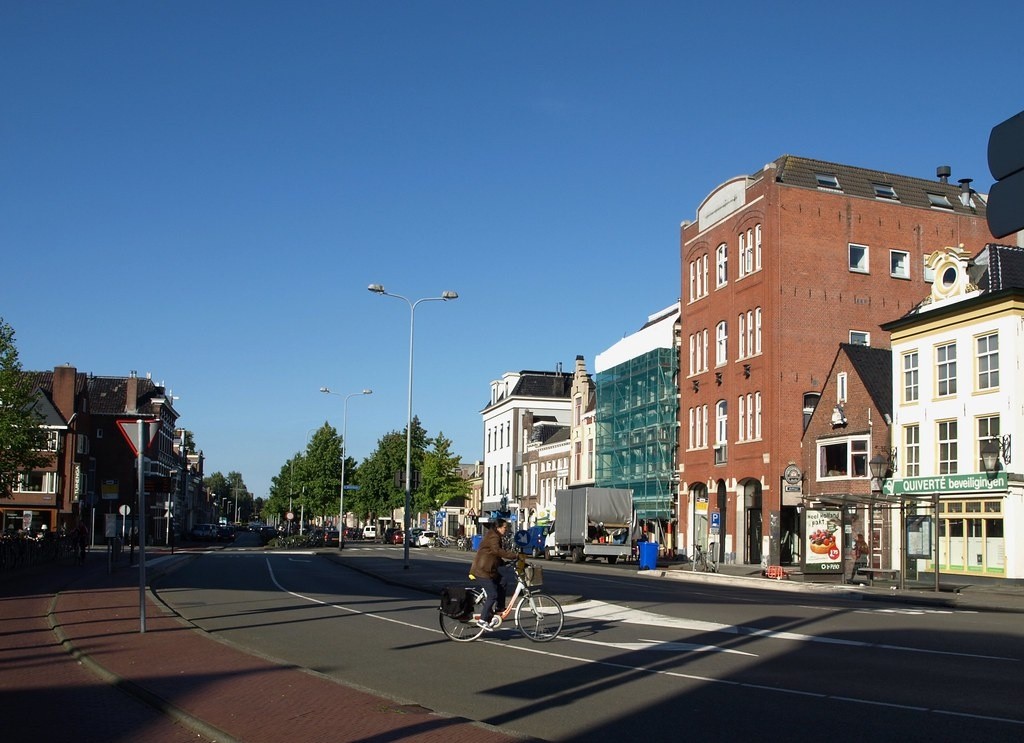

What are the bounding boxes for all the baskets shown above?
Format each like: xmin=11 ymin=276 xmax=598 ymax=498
xmin=514 ymin=566 xmax=543 ymax=586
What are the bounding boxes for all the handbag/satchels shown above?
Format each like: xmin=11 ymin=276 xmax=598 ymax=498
xmin=437 ymin=585 xmax=475 ymax=621
xmin=510 ymin=559 xmax=534 ymax=582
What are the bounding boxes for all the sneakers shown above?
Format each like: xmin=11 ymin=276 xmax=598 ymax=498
xmin=477 ymin=618 xmax=493 ymax=631
xmin=495 ymin=607 xmax=506 ymax=614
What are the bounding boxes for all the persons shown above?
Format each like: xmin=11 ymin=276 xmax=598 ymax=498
xmin=468 ymin=518 xmax=528 ymax=632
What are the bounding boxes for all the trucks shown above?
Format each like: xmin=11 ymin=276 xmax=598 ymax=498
xmin=545 ymin=486 xmax=633 ymax=564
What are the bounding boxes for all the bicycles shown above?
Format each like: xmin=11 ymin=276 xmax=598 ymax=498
xmin=761 ymin=565 xmax=795 ymax=580
xmin=434 ymin=535 xmax=471 ymax=552
xmin=438 ymin=554 xmax=564 ymax=643
xmin=688 ymin=539 xmax=715 ymax=572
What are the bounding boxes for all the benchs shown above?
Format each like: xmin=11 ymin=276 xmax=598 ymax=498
xmin=859 ymin=567 xmax=898 ymax=589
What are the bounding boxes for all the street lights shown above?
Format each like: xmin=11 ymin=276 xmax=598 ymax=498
xmin=319 ymin=385 xmax=374 ymax=546
xmin=367 ymin=282 xmax=459 ymax=567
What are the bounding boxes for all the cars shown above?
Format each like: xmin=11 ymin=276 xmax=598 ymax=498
xmin=191 ymin=522 xmax=276 ymax=543
xmin=515 ymin=526 xmax=551 ymax=558
xmin=320 ymin=523 xmax=439 ymax=547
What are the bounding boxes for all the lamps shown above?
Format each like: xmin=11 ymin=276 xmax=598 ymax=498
xmin=868 ymin=445 xmax=898 ymax=480
xmin=715 ymin=372 xmax=722 ymax=383
xmin=743 ymin=363 xmax=751 ymax=374
xmin=981 ymin=432 xmax=1013 ymax=470
xmin=830 ymin=401 xmax=847 ymax=425
xmin=693 ymin=379 xmax=699 ymax=391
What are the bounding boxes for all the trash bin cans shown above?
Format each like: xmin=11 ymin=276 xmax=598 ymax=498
xmin=471 ymin=534 xmax=482 ymax=551
xmin=637 ymin=542 xmax=659 ymax=569
